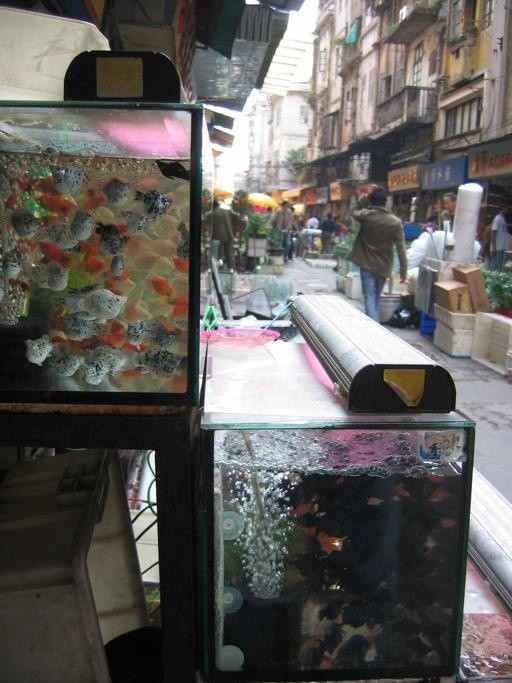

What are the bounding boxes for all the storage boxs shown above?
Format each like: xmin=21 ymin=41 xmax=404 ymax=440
xmin=471 ymin=312 xmax=512 ymax=379
xmin=434 ymin=281 xmax=473 ymax=312
xmin=452 ymin=264 xmax=493 ymax=312
xmin=432 ymin=304 xmax=476 ymax=331
xmin=1 ymin=449 xmax=148 ymax=677
xmin=415 ymin=256 xmax=442 ymax=314
xmin=433 ymin=322 xmax=471 ymax=357
xmin=418 ymin=315 xmax=435 ymax=340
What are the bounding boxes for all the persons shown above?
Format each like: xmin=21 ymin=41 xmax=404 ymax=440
xmin=438 ymin=193 xmax=458 ymax=234
xmin=319 ymin=213 xmax=339 ymax=255
xmin=212 ymin=199 xmax=241 ymax=274
xmin=421 ymin=215 xmax=440 ymax=233
xmin=345 ymin=184 xmax=412 ymax=324
xmin=488 ymin=206 xmax=510 ymax=273
xmin=266 ymin=198 xmax=294 ymax=263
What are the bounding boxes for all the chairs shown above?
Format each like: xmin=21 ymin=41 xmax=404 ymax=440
xmin=205 ymin=248 xmax=275 ymax=322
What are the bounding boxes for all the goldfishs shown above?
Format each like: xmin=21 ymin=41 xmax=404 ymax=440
xmin=1 ymin=159 xmax=189 ymax=392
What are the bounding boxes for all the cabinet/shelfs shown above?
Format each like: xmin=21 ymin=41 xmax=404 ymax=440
xmin=0 ymin=404 xmax=209 ymax=683
